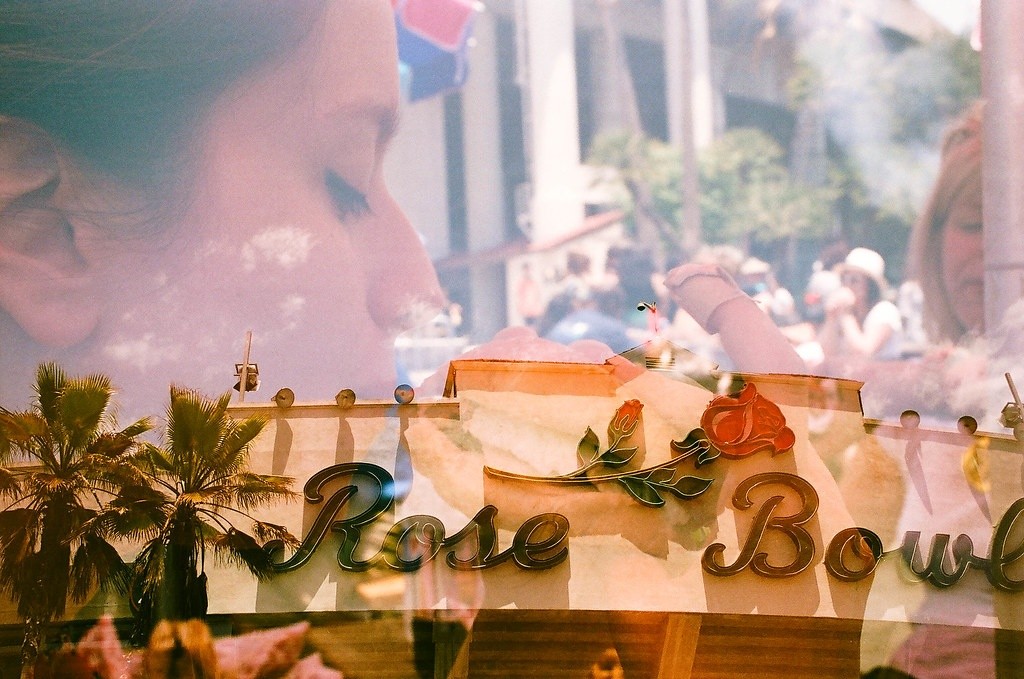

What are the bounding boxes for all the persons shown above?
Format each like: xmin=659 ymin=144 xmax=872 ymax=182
xmin=532 ymin=222 xmax=929 ymax=394
xmin=1 ymin=0 xmax=443 ymax=450
xmin=830 ymin=99 xmax=989 ymax=678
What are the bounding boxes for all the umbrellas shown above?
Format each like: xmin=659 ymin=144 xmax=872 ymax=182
xmin=393 ymin=0 xmax=485 ymax=102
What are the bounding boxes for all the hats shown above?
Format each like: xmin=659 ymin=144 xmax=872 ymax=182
xmin=838 ymin=247 xmax=888 ymax=300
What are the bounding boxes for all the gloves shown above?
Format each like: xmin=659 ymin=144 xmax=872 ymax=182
xmin=663 ymin=264 xmax=757 ymax=332
